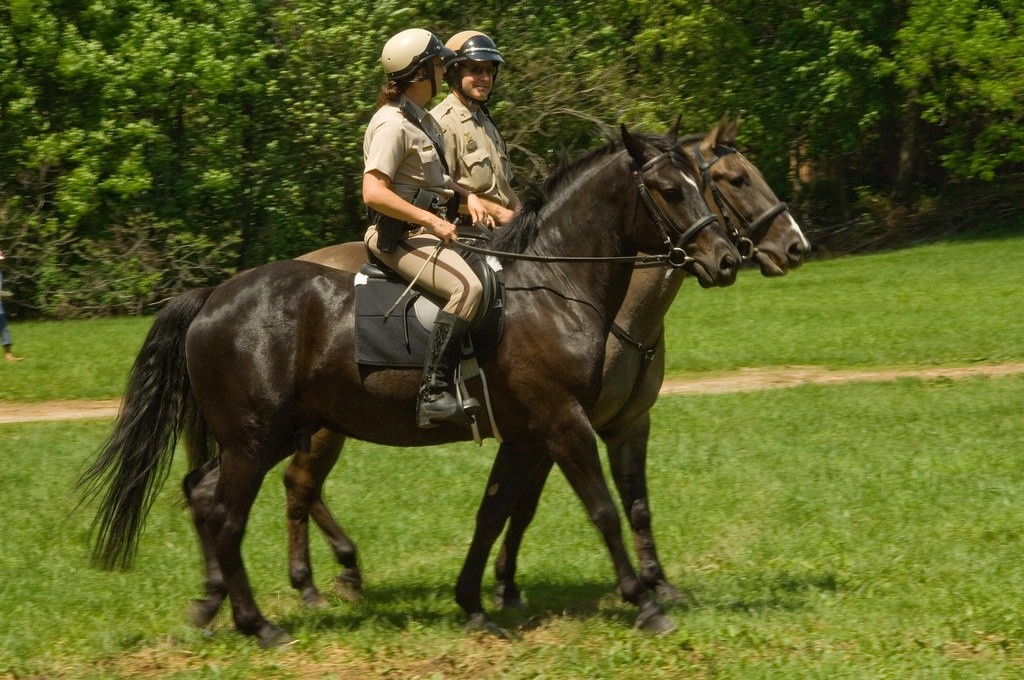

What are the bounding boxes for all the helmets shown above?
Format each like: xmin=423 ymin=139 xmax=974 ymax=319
xmin=381 ymin=28 xmax=457 ymax=81
xmin=441 ymin=30 xmax=505 ymax=84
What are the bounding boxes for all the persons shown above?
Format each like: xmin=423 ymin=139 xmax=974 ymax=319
xmin=363 ymin=29 xmax=497 ymax=430
xmin=430 ymin=31 xmax=528 ymax=229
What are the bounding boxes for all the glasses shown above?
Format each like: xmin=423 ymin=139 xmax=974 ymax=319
xmin=432 ymin=59 xmax=444 ymax=68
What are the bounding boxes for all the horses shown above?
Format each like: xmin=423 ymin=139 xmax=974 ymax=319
xmin=60 ymin=107 xmax=813 ymax=643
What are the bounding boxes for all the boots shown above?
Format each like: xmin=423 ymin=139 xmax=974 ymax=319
xmin=416 ymin=311 xmax=481 ymax=429
